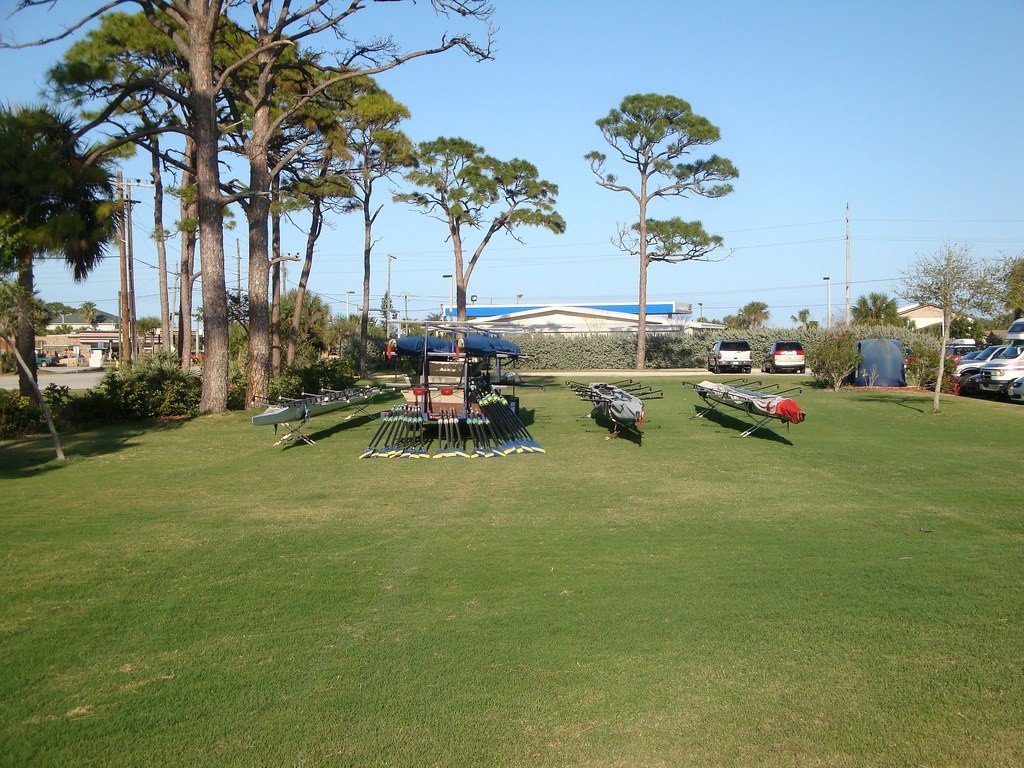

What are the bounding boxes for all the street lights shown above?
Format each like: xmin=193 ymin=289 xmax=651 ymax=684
xmin=697 ymin=303 xmax=703 ymax=323
xmin=443 ymin=273 xmax=454 ymax=328
xmin=822 ymin=277 xmax=832 ymax=332
xmin=516 ymin=294 xmax=523 ymax=304
xmin=346 ymin=290 xmax=356 ymax=321
xmin=471 ymin=294 xmax=477 ymax=305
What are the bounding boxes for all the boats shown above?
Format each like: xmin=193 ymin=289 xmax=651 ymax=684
xmin=584 ymin=381 xmax=647 ymax=424
xmin=252 ymin=386 xmax=397 ymax=428
xmin=694 ymin=378 xmax=807 ymax=425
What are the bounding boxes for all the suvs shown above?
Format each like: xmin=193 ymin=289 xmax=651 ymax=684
xmin=760 ymin=341 xmax=806 ymax=374
xmin=706 ymin=339 xmax=754 ymax=374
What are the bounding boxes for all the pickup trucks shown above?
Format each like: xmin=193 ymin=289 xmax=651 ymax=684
xmin=36 ymin=352 xmax=53 ymax=368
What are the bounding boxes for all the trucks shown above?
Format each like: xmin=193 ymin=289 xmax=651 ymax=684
xmin=975 ymin=316 xmax=1024 ymax=393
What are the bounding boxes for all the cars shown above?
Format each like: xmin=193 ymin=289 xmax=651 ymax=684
xmin=190 ymin=349 xmax=205 ymax=364
xmin=902 ymin=347 xmax=919 ymax=373
xmin=928 ymin=338 xmax=1024 ymax=396
xmin=1007 ymin=375 xmax=1024 ymax=405
